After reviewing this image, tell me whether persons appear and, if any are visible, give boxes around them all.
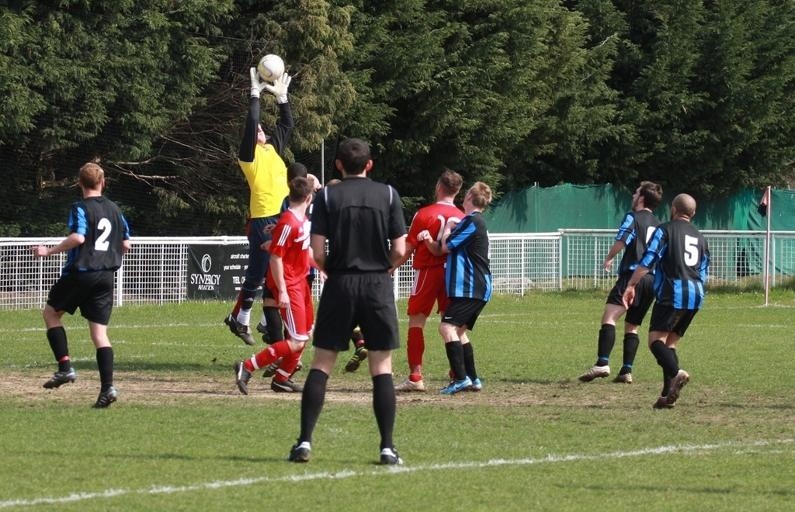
[263,162,317,379]
[32,164,131,410]
[232,178,320,396]
[223,66,295,348]
[622,193,710,409]
[390,170,467,392]
[324,170,368,375]
[578,180,663,384]
[288,136,406,466]
[416,181,492,394]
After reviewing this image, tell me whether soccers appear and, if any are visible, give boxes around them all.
[257,54,285,81]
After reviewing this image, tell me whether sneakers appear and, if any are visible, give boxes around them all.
[271,376,303,393]
[440,376,473,394]
[659,368,690,404]
[379,442,399,465]
[652,396,674,410]
[288,438,313,463]
[468,373,484,391]
[576,365,610,382]
[43,366,77,390]
[234,321,257,347]
[224,314,237,334]
[232,360,252,396]
[613,368,633,385]
[91,384,119,409]
[262,365,277,378]
[344,344,369,372]
[261,333,282,344]
[393,376,427,393]
[255,323,268,333]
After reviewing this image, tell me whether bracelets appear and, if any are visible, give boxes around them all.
[249,86,262,92]
